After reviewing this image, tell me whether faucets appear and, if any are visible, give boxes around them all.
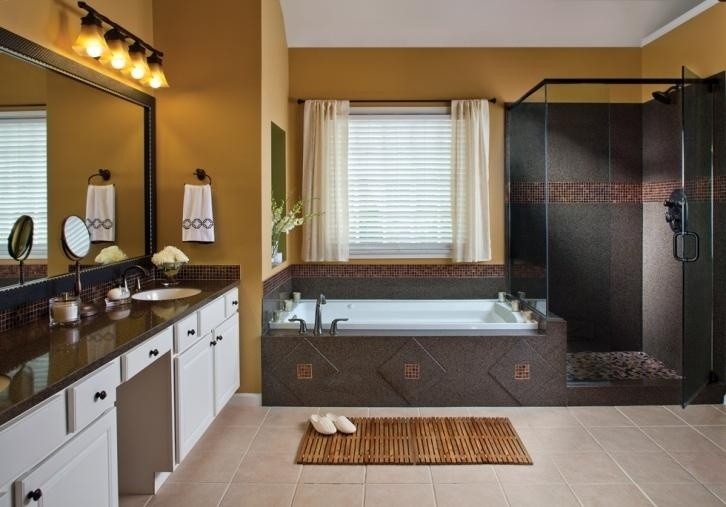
[313,294,326,335]
[122,264,149,294]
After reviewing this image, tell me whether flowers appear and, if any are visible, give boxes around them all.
[272,190,325,255]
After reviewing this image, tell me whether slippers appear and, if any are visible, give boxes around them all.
[326,412,357,433]
[310,414,337,434]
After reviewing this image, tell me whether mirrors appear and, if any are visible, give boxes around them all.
[7,215,33,285]
[61,215,98,317]
[0,26,156,311]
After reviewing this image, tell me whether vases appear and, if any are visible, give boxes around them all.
[271,241,278,267]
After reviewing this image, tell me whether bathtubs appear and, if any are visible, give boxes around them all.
[269,299,539,330]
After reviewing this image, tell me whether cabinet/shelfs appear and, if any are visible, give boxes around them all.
[0,280,240,507]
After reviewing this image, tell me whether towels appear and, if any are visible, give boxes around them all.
[182,183,215,242]
[86,183,116,241]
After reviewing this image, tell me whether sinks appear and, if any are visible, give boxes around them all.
[131,288,202,300]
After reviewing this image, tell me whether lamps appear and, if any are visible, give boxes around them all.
[71,13,113,58]
[98,27,135,75]
[138,52,169,88]
[120,41,153,85]
